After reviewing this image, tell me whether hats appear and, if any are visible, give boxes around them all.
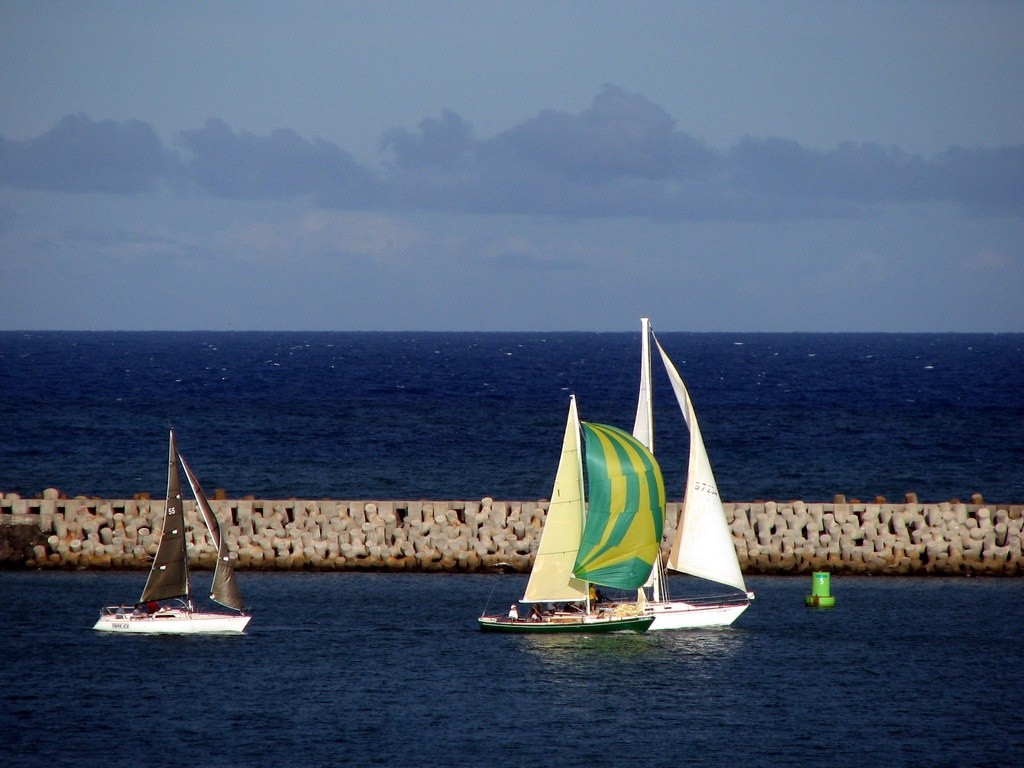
[511,605,516,609]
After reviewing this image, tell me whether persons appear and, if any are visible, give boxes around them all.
[133,604,141,615]
[508,583,612,620]
[117,604,125,614]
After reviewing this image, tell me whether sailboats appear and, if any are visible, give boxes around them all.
[94,427,251,636]
[478,318,756,634]
